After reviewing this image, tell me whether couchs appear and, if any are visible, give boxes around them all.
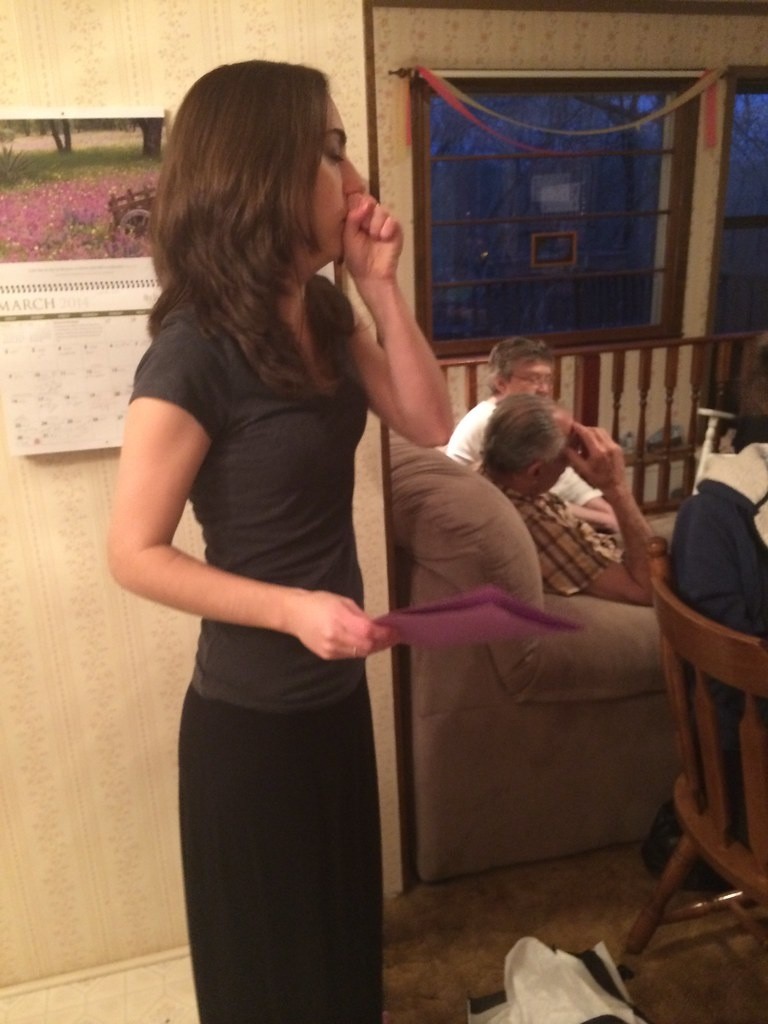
[386,431,687,882]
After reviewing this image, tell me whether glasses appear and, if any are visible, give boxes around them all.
[510,374,557,386]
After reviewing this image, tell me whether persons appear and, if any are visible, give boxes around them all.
[446,335,622,535]
[106,62,455,1023]
[470,392,675,608]
[663,329,768,878]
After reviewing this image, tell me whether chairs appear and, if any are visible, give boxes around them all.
[626,538,767,957]
